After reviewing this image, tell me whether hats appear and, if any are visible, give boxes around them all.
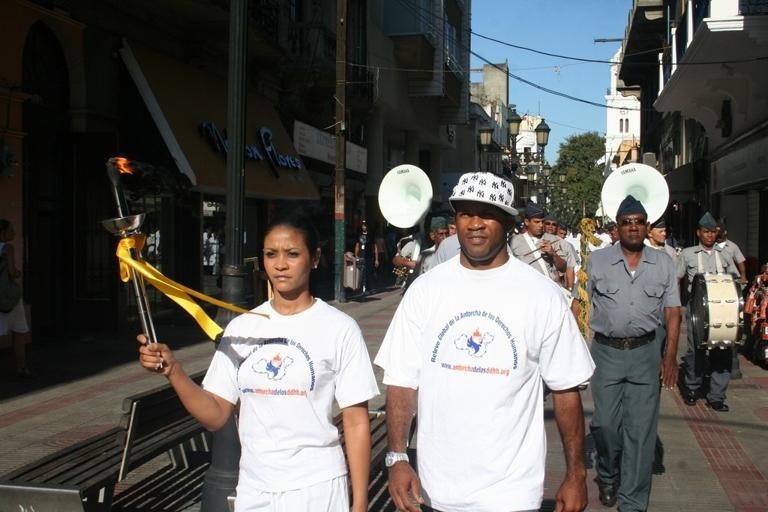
[651,217,666,228]
[617,195,647,217]
[697,211,718,231]
[448,172,520,216]
[525,202,567,230]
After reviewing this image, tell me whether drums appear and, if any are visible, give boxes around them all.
[690,272,748,351]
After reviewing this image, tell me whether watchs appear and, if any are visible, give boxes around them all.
[383,448,411,466]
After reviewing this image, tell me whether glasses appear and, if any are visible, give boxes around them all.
[621,218,644,224]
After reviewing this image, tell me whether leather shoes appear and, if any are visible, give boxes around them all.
[686,391,696,405]
[712,403,728,410]
[601,492,617,506]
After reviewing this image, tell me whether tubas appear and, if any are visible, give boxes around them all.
[601,163,670,226]
[377,163,433,228]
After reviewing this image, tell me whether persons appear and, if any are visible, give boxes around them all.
[136,213,382,511]
[353,218,378,295]
[388,205,749,413]
[371,170,599,511]
[585,195,683,511]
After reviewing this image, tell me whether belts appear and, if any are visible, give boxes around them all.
[593,332,656,350]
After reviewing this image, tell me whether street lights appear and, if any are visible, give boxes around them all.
[475,106,551,181]
[533,161,576,219]
